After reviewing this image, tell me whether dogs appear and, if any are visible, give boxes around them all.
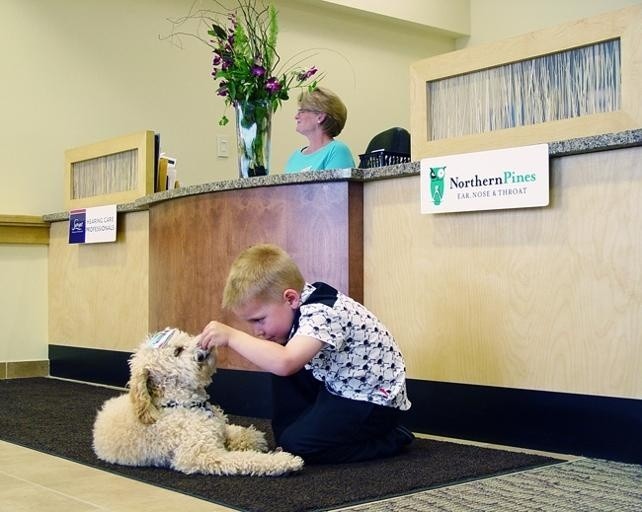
[90,325,305,479]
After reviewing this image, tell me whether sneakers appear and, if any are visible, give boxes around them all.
[378,425,415,455]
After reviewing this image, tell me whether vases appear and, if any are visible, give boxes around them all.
[234,99,270,178]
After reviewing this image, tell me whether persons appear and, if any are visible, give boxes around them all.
[199,245,415,462]
[284,90,355,173]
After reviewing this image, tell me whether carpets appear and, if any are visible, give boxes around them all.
[320,429,642,512]
[0,372,586,512]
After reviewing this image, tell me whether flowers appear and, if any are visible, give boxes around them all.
[157,0,324,175]
